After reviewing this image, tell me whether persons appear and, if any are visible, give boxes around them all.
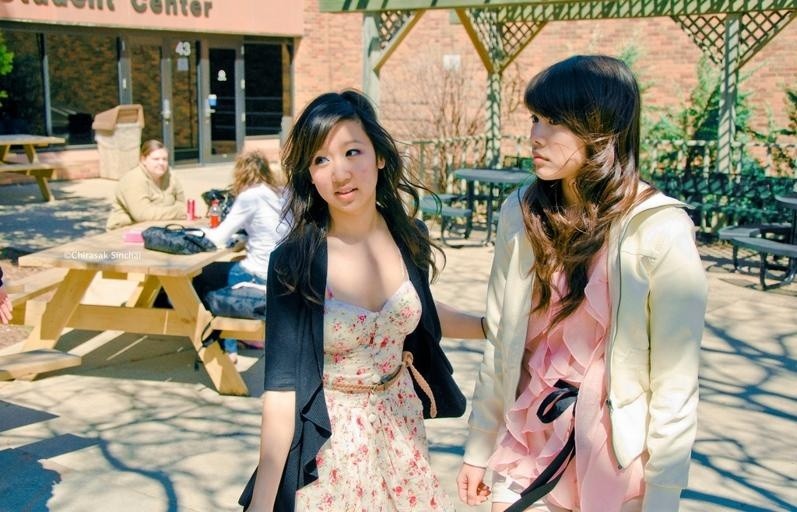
[101,134,190,309]
[183,145,295,369]
[0,266,13,325]
[453,51,711,512]
[236,87,467,511]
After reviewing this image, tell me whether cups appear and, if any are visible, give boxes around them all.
[186,199,194,220]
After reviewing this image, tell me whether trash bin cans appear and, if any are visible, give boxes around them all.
[92,104,145,180]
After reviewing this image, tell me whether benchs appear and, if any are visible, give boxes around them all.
[4,267,72,324]
[719,218,796,289]
[412,192,473,250]
[210,313,265,341]
[1,163,56,177]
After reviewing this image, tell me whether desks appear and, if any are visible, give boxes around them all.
[18,214,252,398]
[452,166,533,249]
[0,134,66,202]
[771,193,796,285]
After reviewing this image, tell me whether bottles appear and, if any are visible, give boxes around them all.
[207,200,220,228]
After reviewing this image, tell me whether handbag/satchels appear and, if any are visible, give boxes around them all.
[141,223,216,255]
[203,281,268,319]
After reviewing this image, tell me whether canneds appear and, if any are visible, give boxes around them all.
[187,199,195,221]
